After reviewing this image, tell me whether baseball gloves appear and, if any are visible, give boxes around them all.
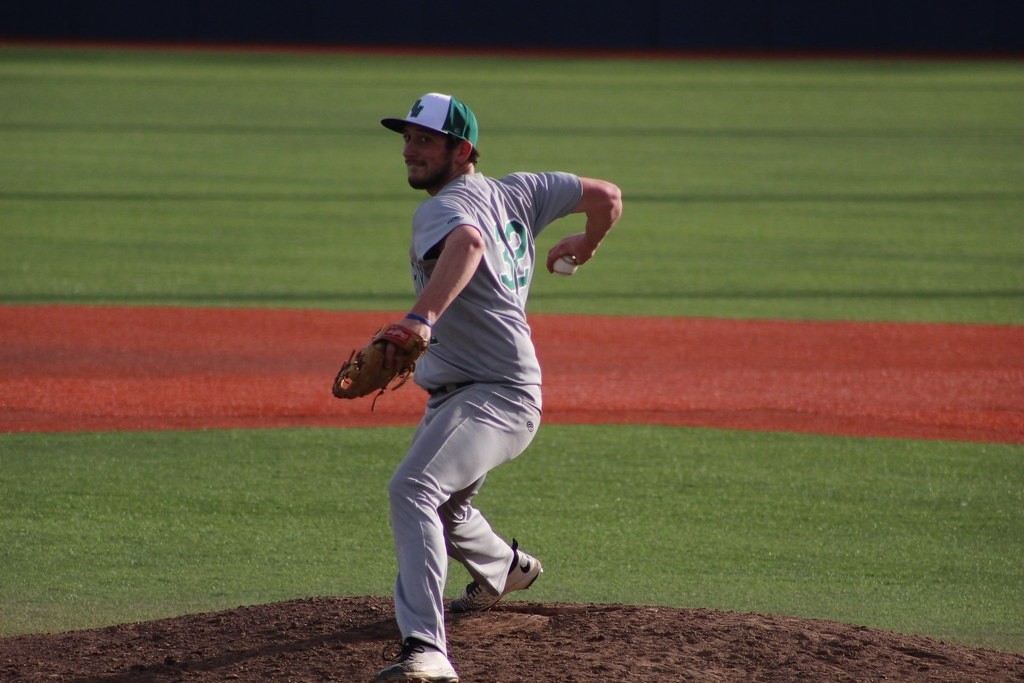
[330,322,429,411]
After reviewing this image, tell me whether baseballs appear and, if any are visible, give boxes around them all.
[553,254,580,278]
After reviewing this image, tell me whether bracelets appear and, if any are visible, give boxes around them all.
[405,314,435,328]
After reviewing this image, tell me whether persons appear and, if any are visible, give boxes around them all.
[331,90,623,683]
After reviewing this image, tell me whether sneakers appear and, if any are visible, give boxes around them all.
[447,539,543,614]
[377,642,459,682]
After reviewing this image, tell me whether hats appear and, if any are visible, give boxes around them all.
[381,91,479,149]
